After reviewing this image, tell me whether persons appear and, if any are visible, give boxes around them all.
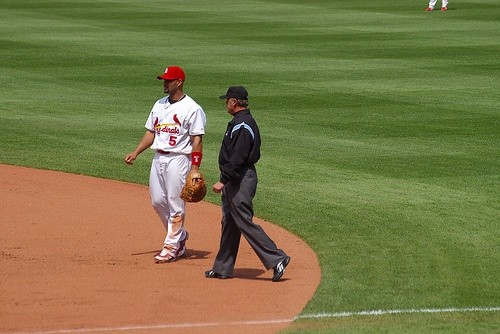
[204,86,291,283]
[425,0,448,11]
[123,65,206,265]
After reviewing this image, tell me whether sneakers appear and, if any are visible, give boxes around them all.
[154,246,182,263]
[272,256,290,281]
[176,231,189,257]
[205,270,227,279]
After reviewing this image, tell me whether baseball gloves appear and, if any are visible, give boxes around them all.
[179,172,207,203]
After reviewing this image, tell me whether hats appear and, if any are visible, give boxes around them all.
[220,86,249,100]
[157,66,185,81]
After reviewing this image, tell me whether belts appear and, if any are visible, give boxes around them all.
[157,150,170,154]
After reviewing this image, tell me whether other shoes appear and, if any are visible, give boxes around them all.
[426,7,435,11]
[441,7,447,11]
